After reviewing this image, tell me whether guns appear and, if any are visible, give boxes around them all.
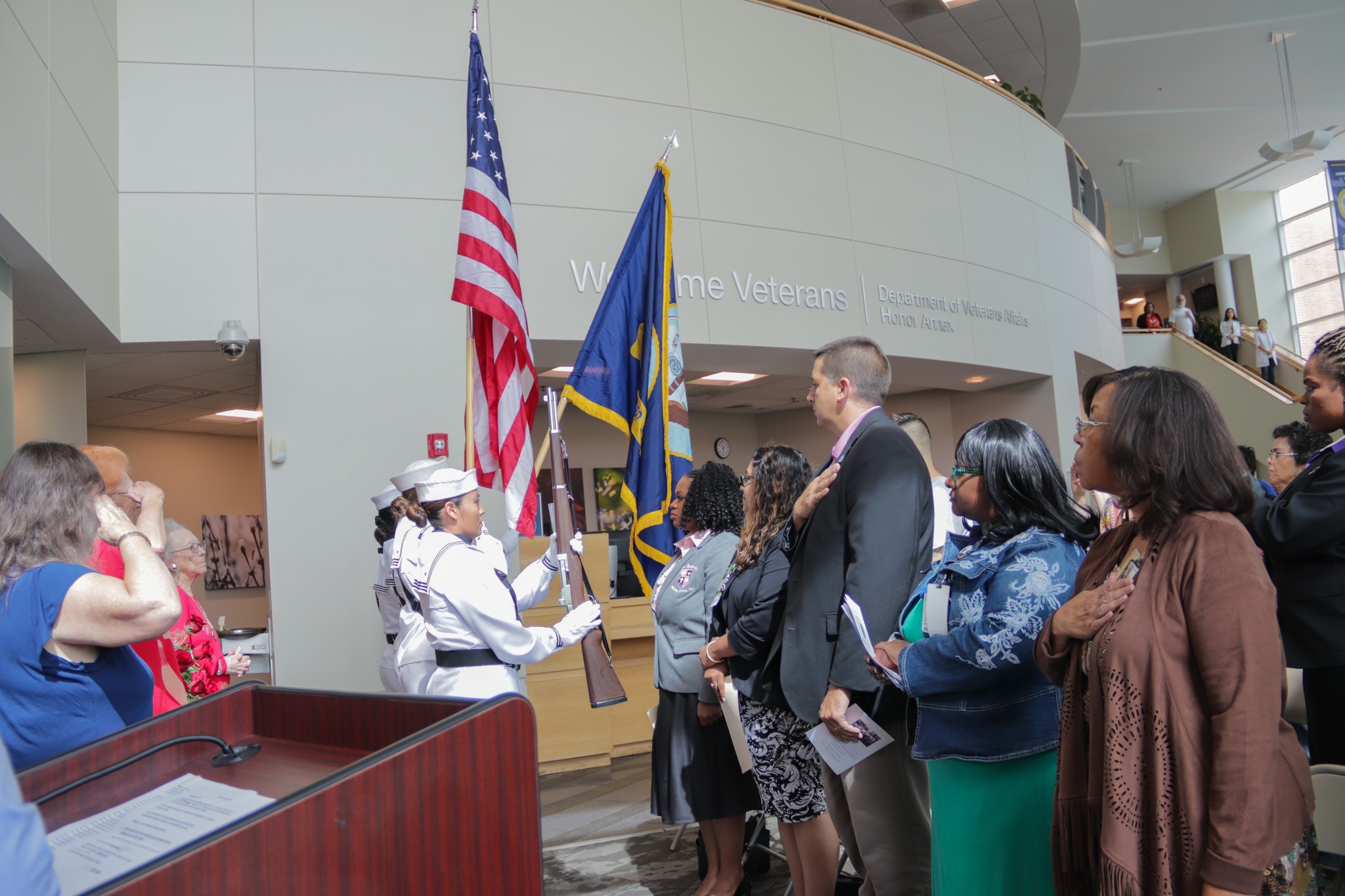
[542,385,630,710]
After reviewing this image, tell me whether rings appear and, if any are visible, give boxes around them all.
[711,683,718,688]
[834,726,840,731]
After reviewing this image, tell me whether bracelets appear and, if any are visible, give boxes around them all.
[117,530,150,548]
[705,639,724,663]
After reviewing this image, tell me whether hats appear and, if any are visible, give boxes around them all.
[390,455,447,492]
[370,484,402,510]
[414,468,479,503]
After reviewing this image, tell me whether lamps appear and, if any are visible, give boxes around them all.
[1257,33,1335,163]
[1113,158,1162,259]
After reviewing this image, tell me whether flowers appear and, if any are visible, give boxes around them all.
[171,614,230,704]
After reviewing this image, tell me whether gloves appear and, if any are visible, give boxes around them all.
[547,532,583,567]
[552,601,602,645]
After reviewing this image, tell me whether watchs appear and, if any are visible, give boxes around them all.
[216,658,223,675]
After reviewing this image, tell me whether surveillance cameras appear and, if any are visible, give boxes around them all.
[215,320,250,361]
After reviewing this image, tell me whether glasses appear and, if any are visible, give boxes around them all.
[952,464,984,483]
[170,540,207,553]
[1076,416,1116,437]
[1266,452,1297,459]
[740,475,757,487]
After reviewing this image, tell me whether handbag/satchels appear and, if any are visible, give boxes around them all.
[695,811,771,882]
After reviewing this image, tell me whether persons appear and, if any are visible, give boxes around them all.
[0,442,182,773]
[1220,307,1243,365]
[1254,319,1280,387]
[73,445,188,718]
[152,515,252,700]
[1136,304,1162,334]
[1168,293,1198,341]
[646,326,1345,896]
[371,457,602,697]
[0,736,60,896]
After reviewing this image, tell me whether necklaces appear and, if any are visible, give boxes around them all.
[175,583,217,639]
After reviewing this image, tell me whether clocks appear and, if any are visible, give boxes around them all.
[714,437,730,459]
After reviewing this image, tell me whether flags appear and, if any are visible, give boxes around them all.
[448,30,541,541]
[564,161,692,608]
